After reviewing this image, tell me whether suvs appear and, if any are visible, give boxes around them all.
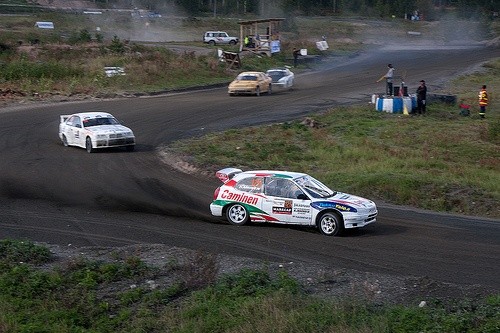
[202,30,239,47]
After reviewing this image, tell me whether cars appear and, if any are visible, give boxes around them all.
[266,67,295,89]
[209,167,379,237]
[227,71,273,97]
[58,111,136,154]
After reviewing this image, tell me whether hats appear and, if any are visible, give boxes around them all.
[419,80,425,82]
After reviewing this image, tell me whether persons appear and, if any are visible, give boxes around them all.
[385,63,395,97]
[293,48,302,67]
[416,80,428,115]
[410,9,419,21]
[267,27,272,39]
[245,36,249,47]
[399,82,409,96]
[479,85,487,118]
